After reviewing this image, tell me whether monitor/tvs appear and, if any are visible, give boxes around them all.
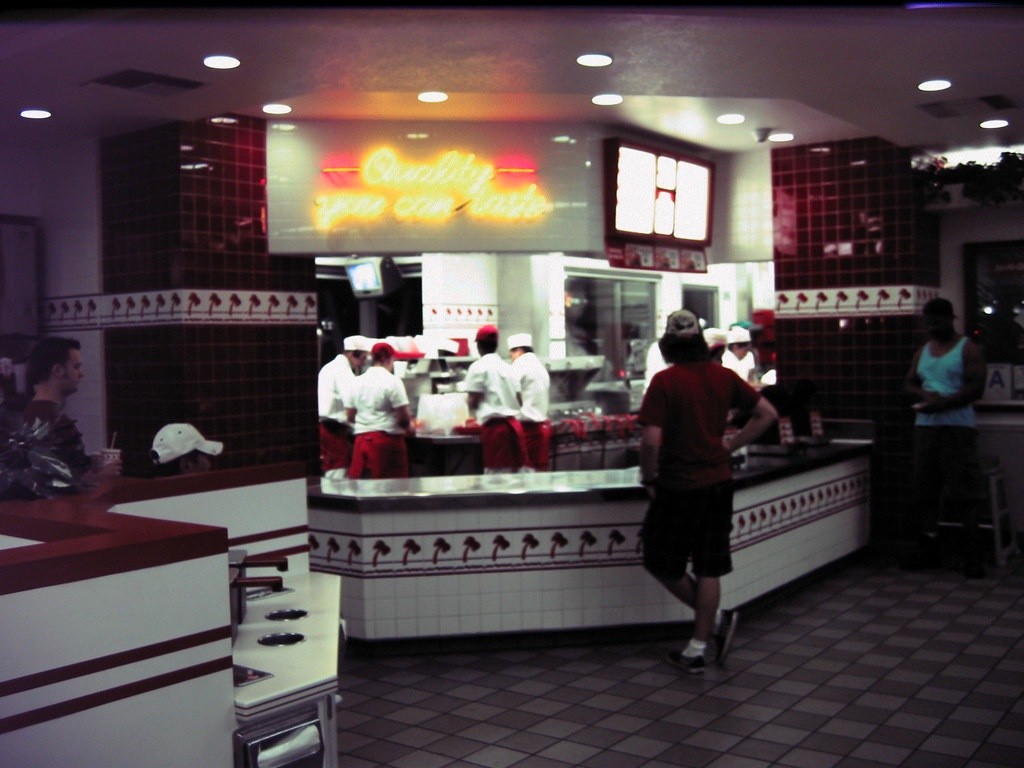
[344,255,401,299]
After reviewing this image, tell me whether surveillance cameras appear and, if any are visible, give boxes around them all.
[752,128,772,143]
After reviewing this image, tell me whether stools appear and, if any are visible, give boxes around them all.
[934,454,1010,563]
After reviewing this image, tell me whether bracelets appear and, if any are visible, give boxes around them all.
[640,471,658,486]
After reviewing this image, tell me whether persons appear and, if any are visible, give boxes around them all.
[463,325,530,474]
[637,319,778,674]
[898,298,987,578]
[319,335,373,470]
[642,320,777,421]
[149,422,223,477]
[343,343,416,479]
[506,334,550,472]
[21,337,122,479]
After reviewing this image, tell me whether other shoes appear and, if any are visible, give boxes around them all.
[713,610,739,664]
[665,645,707,674]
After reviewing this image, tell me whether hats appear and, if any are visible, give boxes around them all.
[149,423,225,464]
[475,325,499,340]
[664,309,700,337]
[702,327,726,350]
[726,325,752,344]
[370,343,394,357]
[922,298,960,320]
[343,334,371,352]
[506,328,534,350]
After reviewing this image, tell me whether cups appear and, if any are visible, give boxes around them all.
[90,448,121,478]
